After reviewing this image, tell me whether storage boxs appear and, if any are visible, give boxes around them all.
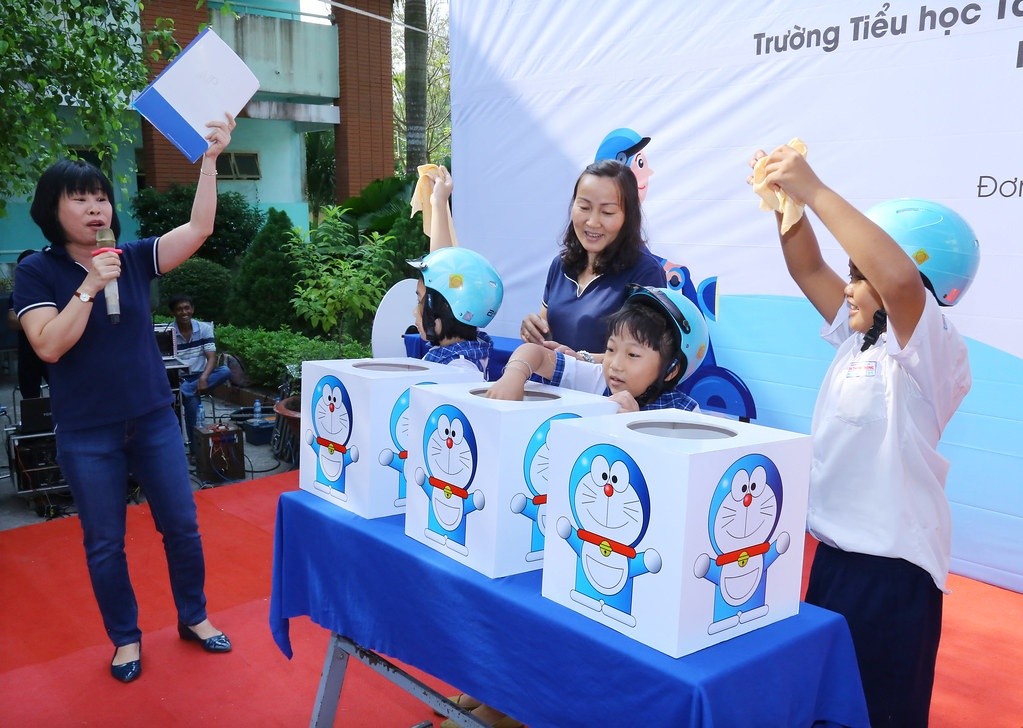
[541,407,815,657]
[403,379,621,580]
[300,356,484,519]
[245,417,276,446]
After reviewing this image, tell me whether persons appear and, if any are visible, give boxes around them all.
[8,250,49,398]
[406,165,524,728]
[483,284,709,413]
[167,295,232,463]
[520,160,665,363]
[13,112,236,683]
[747,145,981,728]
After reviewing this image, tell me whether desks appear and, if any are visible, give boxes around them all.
[271,489,872,728]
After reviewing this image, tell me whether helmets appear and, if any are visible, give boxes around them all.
[624,283,710,390]
[862,198,981,308]
[405,246,504,328]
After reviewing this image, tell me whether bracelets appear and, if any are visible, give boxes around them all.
[502,366,527,381]
[505,359,532,380]
[579,351,594,363]
[201,169,218,176]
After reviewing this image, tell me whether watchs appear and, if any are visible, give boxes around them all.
[74,292,94,303]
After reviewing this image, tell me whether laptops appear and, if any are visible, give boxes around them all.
[15,398,53,434]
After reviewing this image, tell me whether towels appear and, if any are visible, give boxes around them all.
[409,163,460,247]
[753,136,808,236]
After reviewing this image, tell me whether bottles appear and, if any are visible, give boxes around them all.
[253,399,261,421]
[196,405,205,429]
[275,397,281,404]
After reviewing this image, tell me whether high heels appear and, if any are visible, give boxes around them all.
[177,619,232,652]
[111,639,140,683]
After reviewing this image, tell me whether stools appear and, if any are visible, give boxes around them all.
[0,405,13,480]
[201,393,216,424]
[12,384,49,425]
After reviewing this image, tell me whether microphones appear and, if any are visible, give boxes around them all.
[95,228,121,326]
